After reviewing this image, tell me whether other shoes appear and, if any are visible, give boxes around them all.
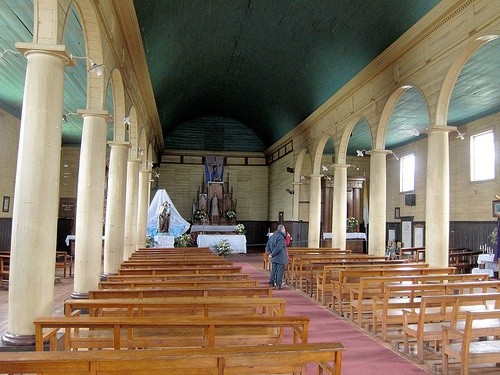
[269,287,283,290]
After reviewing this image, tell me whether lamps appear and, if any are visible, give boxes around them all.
[322,164,331,171]
[301,175,306,180]
[287,167,295,173]
[457,129,466,140]
[146,159,160,178]
[70,54,106,77]
[138,147,144,156]
[123,115,131,126]
[63,113,79,123]
[356,149,366,156]
[352,165,359,172]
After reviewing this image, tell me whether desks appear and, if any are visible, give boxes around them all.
[323,233,366,253]
[196,234,247,254]
[191,225,237,241]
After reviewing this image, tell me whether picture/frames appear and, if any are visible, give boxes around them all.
[395,207,401,219]
[3,196,10,212]
[492,200,500,217]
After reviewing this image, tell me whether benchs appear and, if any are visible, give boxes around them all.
[65,235,105,260]
[0,247,500,375]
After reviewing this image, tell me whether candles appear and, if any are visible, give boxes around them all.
[480,244,493,254]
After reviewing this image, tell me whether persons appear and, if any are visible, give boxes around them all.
[272,223,290,247]
[266,224,288,289]
[212,192,219,215]
[157,201,174,234]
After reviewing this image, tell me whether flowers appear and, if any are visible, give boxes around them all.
[347,217,358,229]
[235,224,245,234]
[175,234,194,247]
[224,210,236,220]
[195,209,207,221]
[211,239,233,256]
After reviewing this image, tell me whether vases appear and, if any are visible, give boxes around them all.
[349,227,353,233]
